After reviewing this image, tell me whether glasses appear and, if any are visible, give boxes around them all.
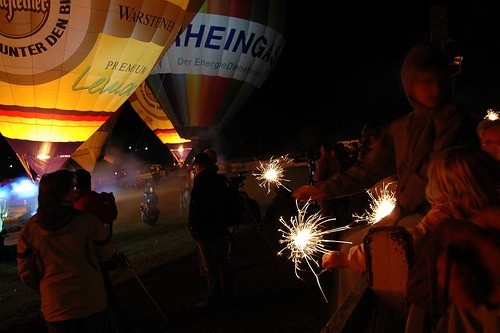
[64,186,77,193]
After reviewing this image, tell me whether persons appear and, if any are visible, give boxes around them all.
[17,169,116,333]
[291,36,500,333]
[68,168,118,224]
[187,148,233,308]
[263,185,295,222]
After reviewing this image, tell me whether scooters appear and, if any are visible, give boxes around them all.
[140,196,159,226]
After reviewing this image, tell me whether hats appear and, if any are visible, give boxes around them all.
[190,152,212,166]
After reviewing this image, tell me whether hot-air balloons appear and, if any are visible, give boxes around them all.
[143,0,286,181]
[126,80,197,174]
[0,0,206,188]
